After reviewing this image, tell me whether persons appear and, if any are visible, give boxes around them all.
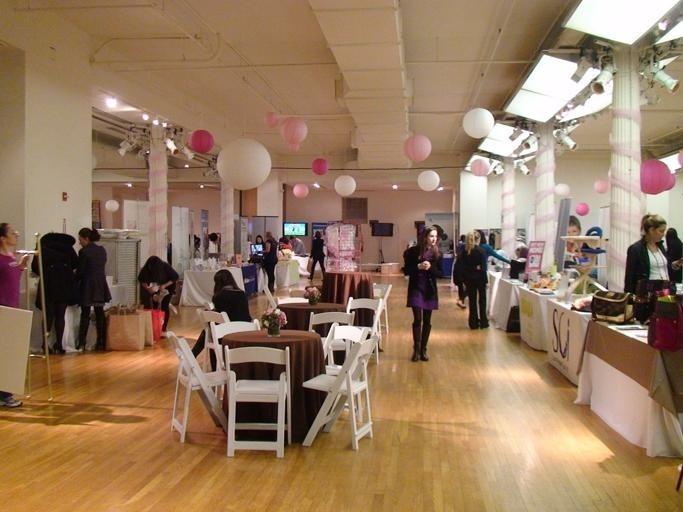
[138,256,180,332]
[255,232,278,292]
[476,229,511,272]
[665,227,683,282]
[453,234,468,308]
[255,234,265,248]
[0,222,30,408]
[567,215,581,236]
[75,227,112,351]
[460,230,490,329]
[403,226,444,362]
[191,269,252,372]
[31,232,78,354]
[307,231,326,281]
[624,213,683,324]
[290,235,306,255]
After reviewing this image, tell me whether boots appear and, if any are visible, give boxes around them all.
[76,326,88,350]
[95,328,103,350]
[412,324,420,361]
[421,325,431,360]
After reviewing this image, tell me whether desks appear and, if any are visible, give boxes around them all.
[278,302,349,365]
[494,277,526,332]
[323,271,374,327]
[586,319,679,429]
[223,331,330,443]
[547,298,590,387]
[517,284,559,351]
[183,263,266,307]
[275,259,299,288]
[488,269,502,319]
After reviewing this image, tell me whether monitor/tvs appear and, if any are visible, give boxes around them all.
[283,222,308,236]
[249,243,264,257]
[371,222,394,236]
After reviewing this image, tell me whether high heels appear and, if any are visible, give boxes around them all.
[53,343,65,354]
[308,277,313,281]
[42,344,51,354]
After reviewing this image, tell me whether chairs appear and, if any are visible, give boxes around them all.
[209,319,261,408]
[195,308,231,401]
[325,322,369,433]
[223,345,293,458]
[302,334,381,451]
[373,281,392,351]
[307,311,356,352]
[165,331,237,444]
[345,297,383,365]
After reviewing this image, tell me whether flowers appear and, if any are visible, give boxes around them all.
[303,287,323,306]
[261,309,288,336]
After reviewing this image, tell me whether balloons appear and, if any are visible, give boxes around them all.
[555,183,570,197]
[594,180,608,193]
[641,158,675,194]
[462,108,494,139]
[470,158,490,177]
[311,158,328,175]
[217,136,272,191]
[404,134,432,162]
[417,170,440,191]
[280,118,308,152]
[334,175,356,197]
[576,202,589,216]
[264,111,279,128]
[189,129,214,153]
[293,183,309,199]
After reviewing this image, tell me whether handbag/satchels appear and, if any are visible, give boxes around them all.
[648,290,683,351]
[589,292,636,326]
[106,303,144,351]
[134,311,155,347]
[143,297,165,343]
[248,252,264,264]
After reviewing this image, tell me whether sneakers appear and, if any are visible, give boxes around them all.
[0,396,22,407]
[456,301,466,309]
[480,324,489,329]
